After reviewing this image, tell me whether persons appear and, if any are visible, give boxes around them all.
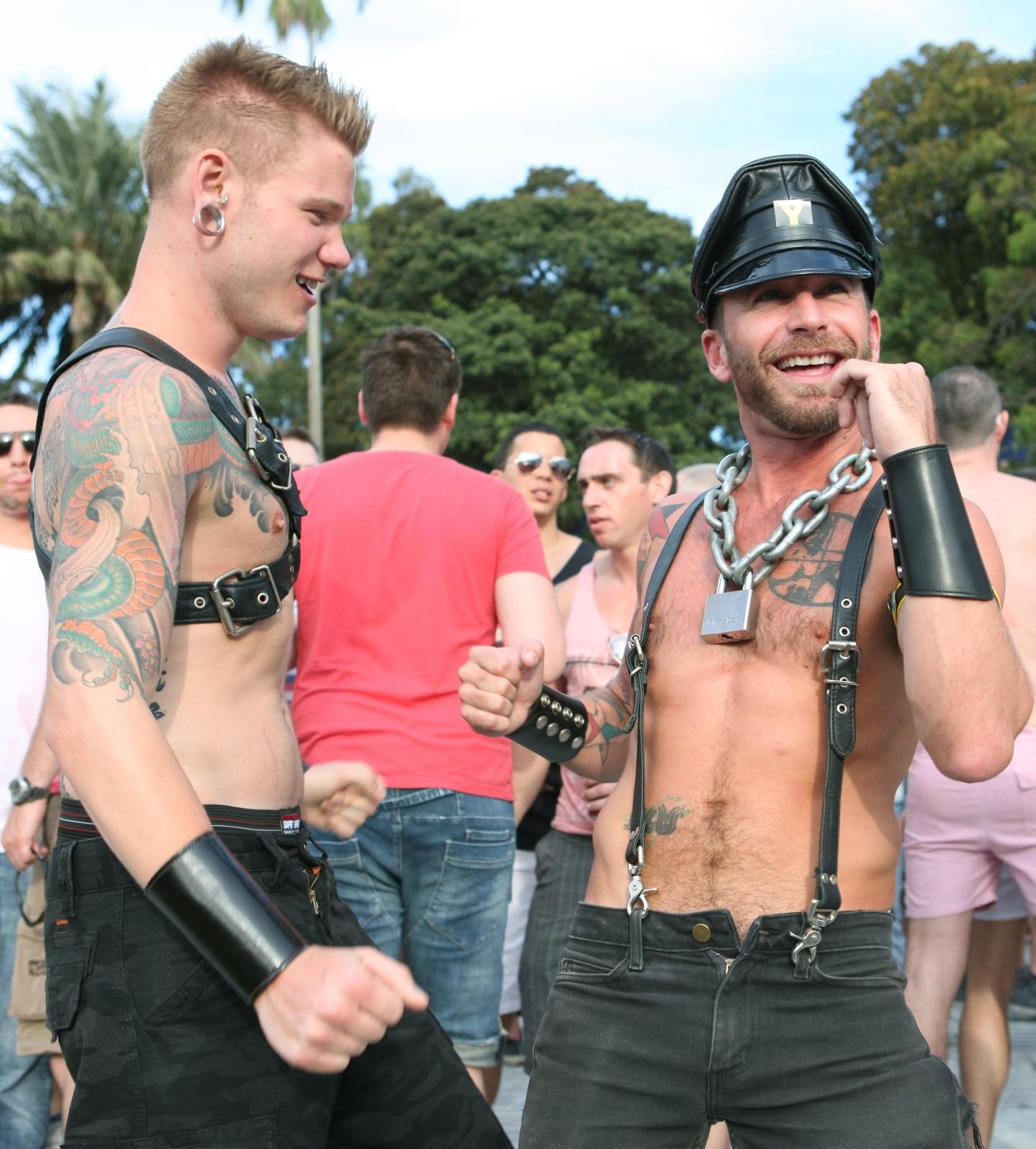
[459,156,1035,1149]
[0,326,1036,1149]
[30,41,516,1149]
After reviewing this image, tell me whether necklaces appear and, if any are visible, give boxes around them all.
[698,443,879,647]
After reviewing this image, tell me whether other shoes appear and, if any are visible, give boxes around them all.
[45,1114,63,1146]
[951,959,1036,1021]
[501,1028,525,1067]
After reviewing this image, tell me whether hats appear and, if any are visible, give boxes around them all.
[691,154,885,328]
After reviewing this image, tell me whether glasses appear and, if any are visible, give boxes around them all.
[0,429,33,454]
[507,452,573,482]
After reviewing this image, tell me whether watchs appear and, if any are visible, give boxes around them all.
[8,774,49,806]
